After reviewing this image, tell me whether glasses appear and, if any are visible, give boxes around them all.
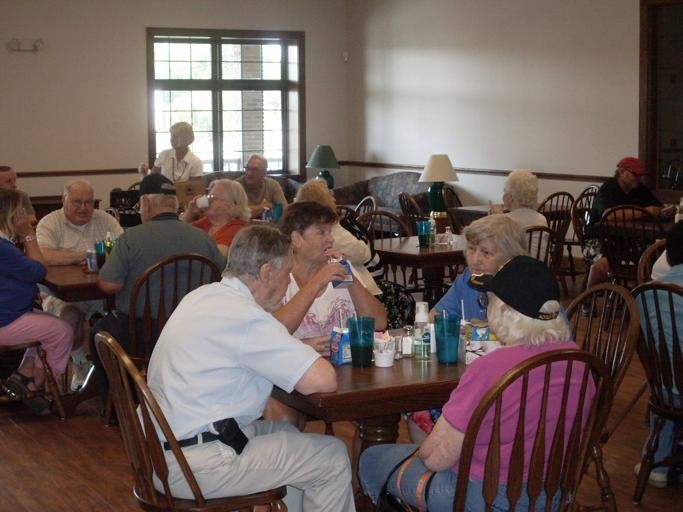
[626,169,640,177]
[243,167,266,174]
[207,195,235,204]
[477,293,489,309]
[72,199,93,208]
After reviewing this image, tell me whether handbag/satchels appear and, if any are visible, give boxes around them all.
[376,448,436,512]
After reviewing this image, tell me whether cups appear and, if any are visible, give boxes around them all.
[373,348,395,367]
[95,241,106,270]
[196,195,209,208]
[347,316,375,369]
[416,221,432,248]
[437,233,450,245]
[433,314,461,363]
[86,250,97,271]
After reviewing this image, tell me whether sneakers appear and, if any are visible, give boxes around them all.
[634,463,668,488]
[71,361,90,391]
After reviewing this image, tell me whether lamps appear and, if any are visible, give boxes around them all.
[305,144,341,190]
[417,155,459,218]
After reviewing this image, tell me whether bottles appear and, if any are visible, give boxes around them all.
[444,226,452,245]
[401,326,415,358]
[414,302,431,362]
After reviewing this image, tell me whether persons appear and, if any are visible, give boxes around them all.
[232,156,287,218]
[136,224,356,508]
[404,214,575,445]
[581,158,671,307]
[488,170,548,261]
[0,165,37,250]
[179,178,249,259]
[264,203,388,444]
[359,257,595,507]
[2,190,72,417]
[95,172,224,424]
[634,224,683,488]
[140,120,201,198]
[37,183,125,391]
[295,177,413,330]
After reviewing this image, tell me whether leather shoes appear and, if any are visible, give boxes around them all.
[582,303,597,317]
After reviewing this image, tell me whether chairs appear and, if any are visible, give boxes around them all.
[102,253,221,426]
[523,226,563,273]
[335,206,355,225]
[625,283,683,504]
[355,196,377,232]
[552,184,599,283]
[95,330,292,512]
[454,348,615,512]
[442,184,463,236]
[398,190,425,234]
[600,204,666,332]
[0,340,68,421]
[566,283,640,512]
[572,192,603,299]
[356,210,419,304]
[103,207,119,225]
[537,192,575,295]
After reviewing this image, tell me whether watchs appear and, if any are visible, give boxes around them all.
[18,235,36,243]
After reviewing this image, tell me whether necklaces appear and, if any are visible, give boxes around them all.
[171,155,187,184]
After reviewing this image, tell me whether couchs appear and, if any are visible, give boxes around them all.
[200,171,304,206]
[335,172,463,239]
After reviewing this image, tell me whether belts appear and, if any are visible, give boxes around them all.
[163,432,221,451]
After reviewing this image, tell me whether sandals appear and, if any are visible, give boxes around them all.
[6,370,50,413]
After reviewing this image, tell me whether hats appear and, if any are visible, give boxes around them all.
[617,158,645,174]
[468,256,561,320]
[130,173,177,205]
